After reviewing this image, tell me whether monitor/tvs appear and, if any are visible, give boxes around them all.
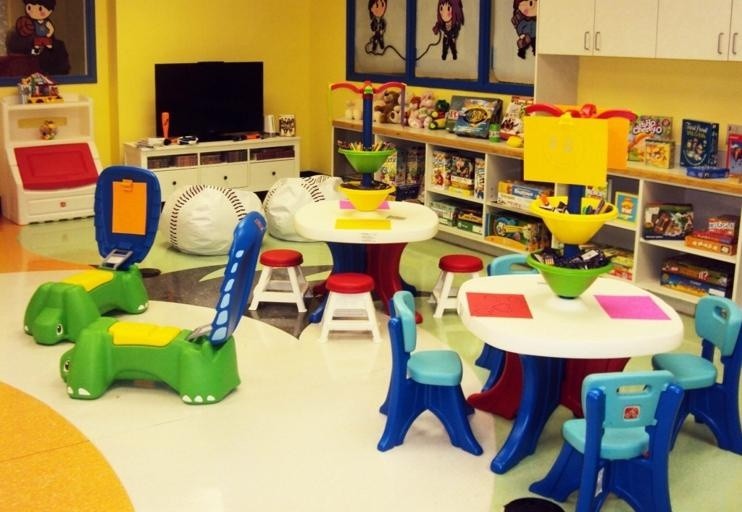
[155,61,264,142]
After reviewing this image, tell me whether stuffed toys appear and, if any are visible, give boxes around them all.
[345,91,450,130]
